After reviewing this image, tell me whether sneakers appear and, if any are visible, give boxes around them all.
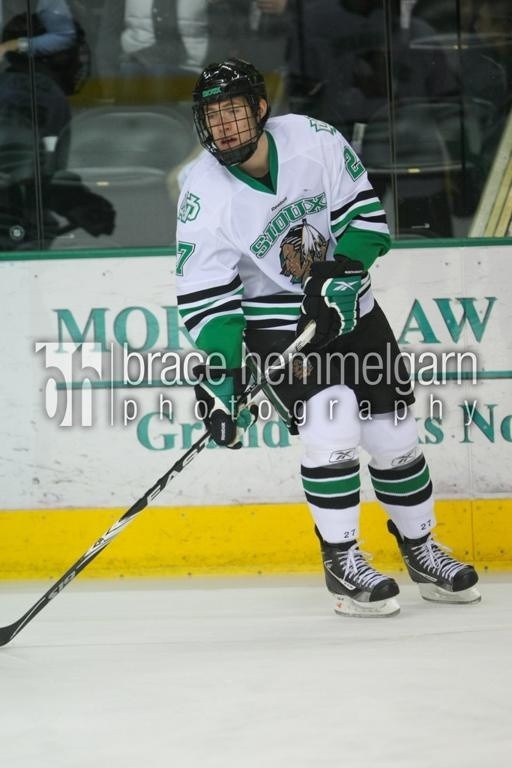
[323,540,399,603]
[404,533,478,591]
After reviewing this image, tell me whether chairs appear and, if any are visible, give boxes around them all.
[0,2,512,254]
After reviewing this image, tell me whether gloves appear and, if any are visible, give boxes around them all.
[297,254,371,349]
[193,365,258,449]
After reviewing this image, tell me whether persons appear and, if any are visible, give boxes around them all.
[1,1,512,252]
[172,59,481,602]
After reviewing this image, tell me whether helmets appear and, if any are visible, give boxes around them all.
[192,57,271,167]
